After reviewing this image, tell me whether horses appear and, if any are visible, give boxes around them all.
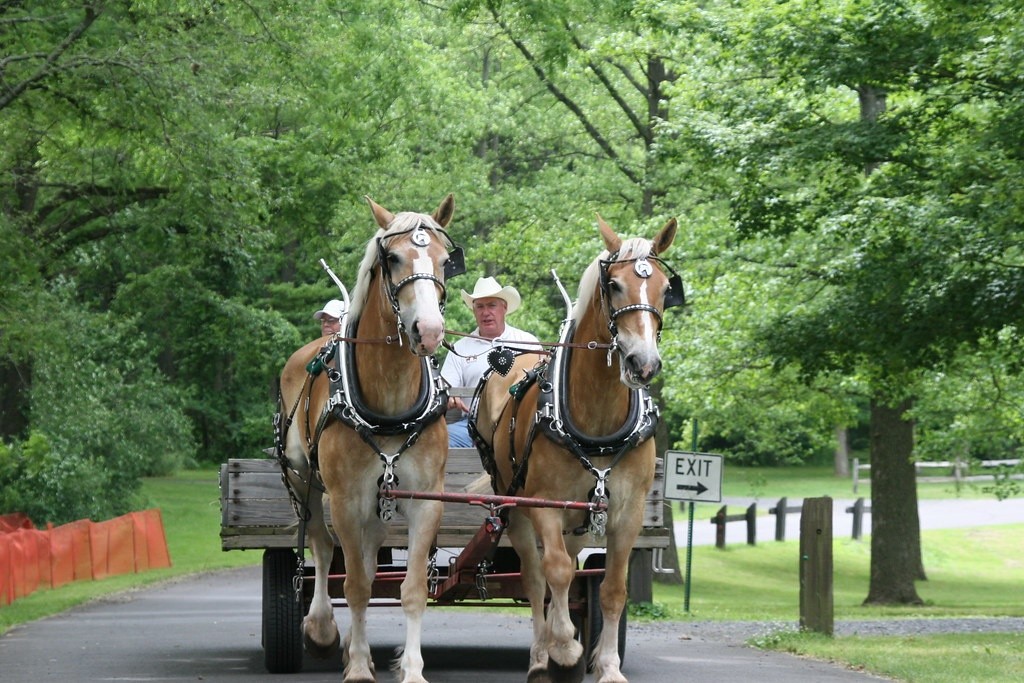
[278,195,455,683]
[469,211,678,683]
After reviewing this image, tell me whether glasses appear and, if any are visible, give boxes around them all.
[320,317,337,325]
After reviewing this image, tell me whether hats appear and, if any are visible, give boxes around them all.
[460,276,521,316]
[313,300,344,319]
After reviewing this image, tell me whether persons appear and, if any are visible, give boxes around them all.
[435,276,541,446]
[313,299,346,337]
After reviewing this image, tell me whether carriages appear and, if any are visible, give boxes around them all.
[217,194,682,664]
